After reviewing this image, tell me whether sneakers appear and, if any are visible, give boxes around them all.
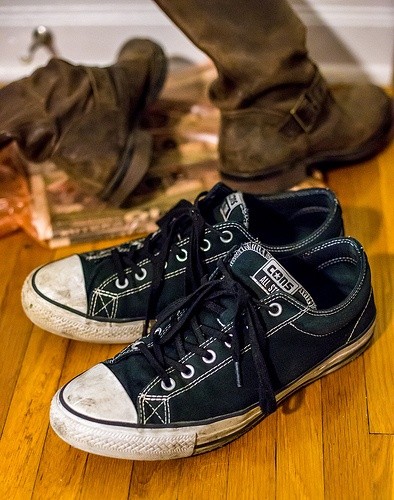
[21,181,345,344]
[49,237,377,461]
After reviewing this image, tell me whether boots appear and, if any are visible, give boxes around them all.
[0,39,166,210]
[156,0,393,194]
